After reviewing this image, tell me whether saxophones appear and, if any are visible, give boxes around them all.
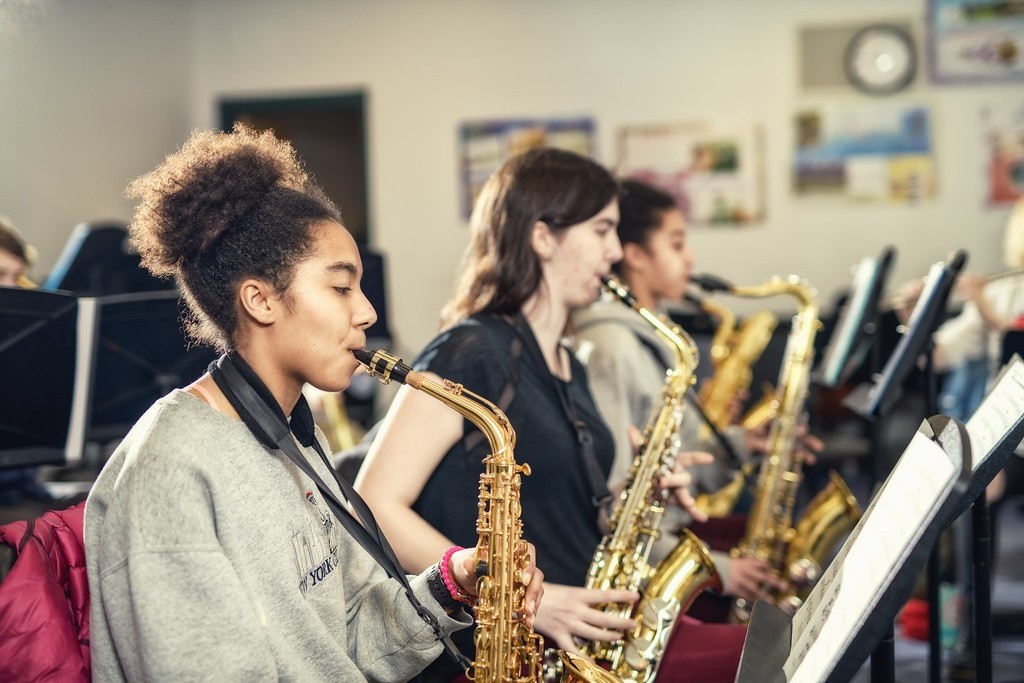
[681,290,780,518]
[687,271,865,627]
[570,273,727,683]
[351,346,621,683]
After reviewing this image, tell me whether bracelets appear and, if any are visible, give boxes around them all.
[440,547,478,607]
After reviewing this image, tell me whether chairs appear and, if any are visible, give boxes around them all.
[0,501,93,683]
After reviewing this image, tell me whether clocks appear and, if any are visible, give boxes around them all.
[842,24,916,97]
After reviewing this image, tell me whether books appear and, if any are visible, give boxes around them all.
[782,361,1024,683]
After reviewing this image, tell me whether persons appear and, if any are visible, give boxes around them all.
[558,178,826,607]
[0,218,36,289]
[345,146,713,665]
[83,123,543,683]
[826,202,1024,644]
